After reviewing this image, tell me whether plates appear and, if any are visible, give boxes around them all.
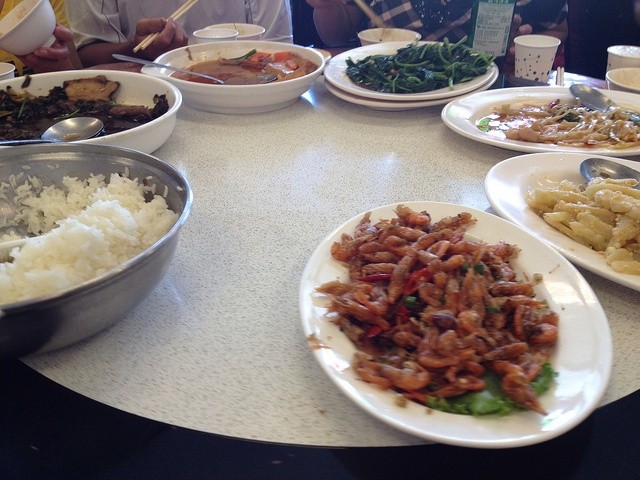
[326,41,496,100]
[327,56,501,112]
[300,201,612,446]
[484,151,640,290]
[439,85,638,155]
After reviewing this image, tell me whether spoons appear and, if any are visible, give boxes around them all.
[0,117,103,147]
[579,158,639,195]
[570,84,640,123]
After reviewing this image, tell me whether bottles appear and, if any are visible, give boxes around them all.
[466,0,516,71]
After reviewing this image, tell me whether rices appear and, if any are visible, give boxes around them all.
[0,160,183,304]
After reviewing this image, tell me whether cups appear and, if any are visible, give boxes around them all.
[193,29,238,40]
[513,35,560,83]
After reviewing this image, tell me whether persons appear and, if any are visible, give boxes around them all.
[1,0,85,73]
[64,0,294,68]
[305,0,570,66]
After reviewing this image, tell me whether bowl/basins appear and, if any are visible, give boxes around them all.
[152,39,324,115]
[358,27,420,41]
[0,69,182,155]
[603,67,638,93]
[0,143,193,360]
[210,22,266,40]
[1,1,58,55]
[0,62,16,80]
[609,44,640,67]
